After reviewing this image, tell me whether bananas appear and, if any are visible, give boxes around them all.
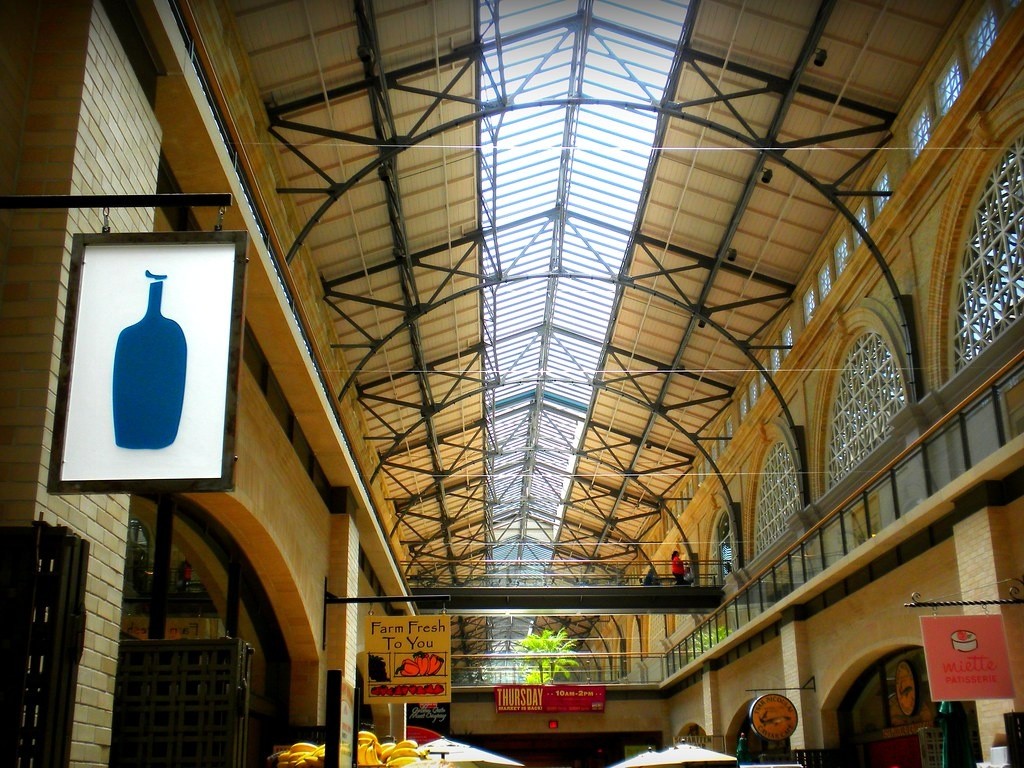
[357,730,431,768]
[276,742,326,768]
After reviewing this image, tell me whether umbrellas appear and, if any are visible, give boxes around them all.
[736,732,751,762]
[418,738,524,766]
[938,701,975,768]
[609,738,737,768]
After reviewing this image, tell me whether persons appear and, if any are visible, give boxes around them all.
[643,569,659,586]
[671,550,683,583]
[675,567,693,586]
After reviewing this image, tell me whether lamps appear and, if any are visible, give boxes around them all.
[762,167,773,183]
[393,247,405,262]
[356,44,373,61]
[587,516,617,573]
[646,442,652,449]
[813,47,828,66]
[622,493,629,501]
[698,320,706,327]
[727,247,737,261]
[378,164,392,182]
